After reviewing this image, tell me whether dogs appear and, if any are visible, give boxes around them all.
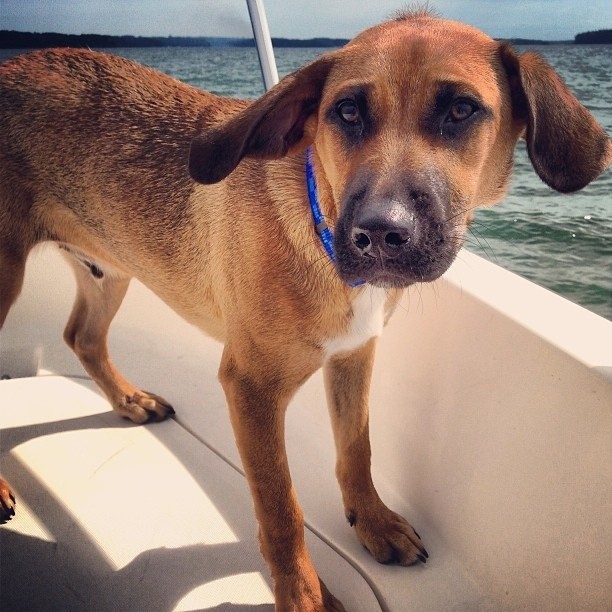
[0,1,611,612]
